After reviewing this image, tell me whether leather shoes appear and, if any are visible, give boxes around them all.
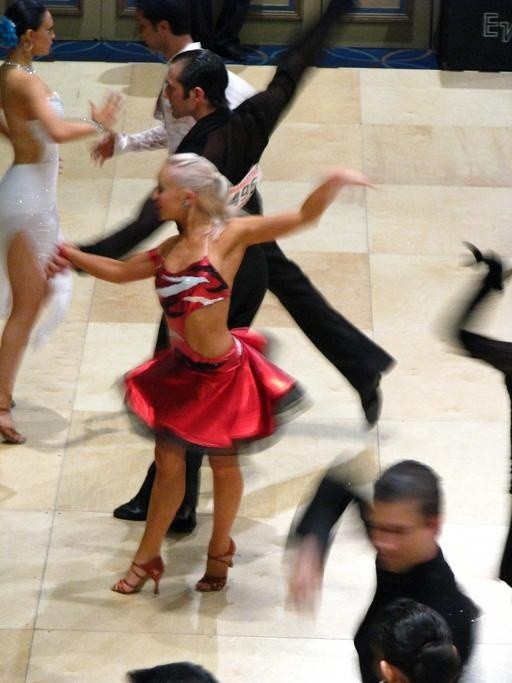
[170,471,198,532]
[113,461,157,521]
[357,357,393,423]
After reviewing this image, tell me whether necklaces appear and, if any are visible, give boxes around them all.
[4,57,37,79]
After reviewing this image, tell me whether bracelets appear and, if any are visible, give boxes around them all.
[90,120,104,129]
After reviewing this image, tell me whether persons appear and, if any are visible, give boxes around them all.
[46,0,365,537]
[452,240,512,588]
[86,0,397,427]
[55,150,380,598]
[1,0,133,451]
[280,445,481,682]
[368,594,462,681]
[170,0,257,64]
[127,660,219,683]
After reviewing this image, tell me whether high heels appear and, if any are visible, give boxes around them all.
[195,536,236,591]
[111,556,164,594]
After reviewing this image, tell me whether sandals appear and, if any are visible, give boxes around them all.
[0,407,27,444]
